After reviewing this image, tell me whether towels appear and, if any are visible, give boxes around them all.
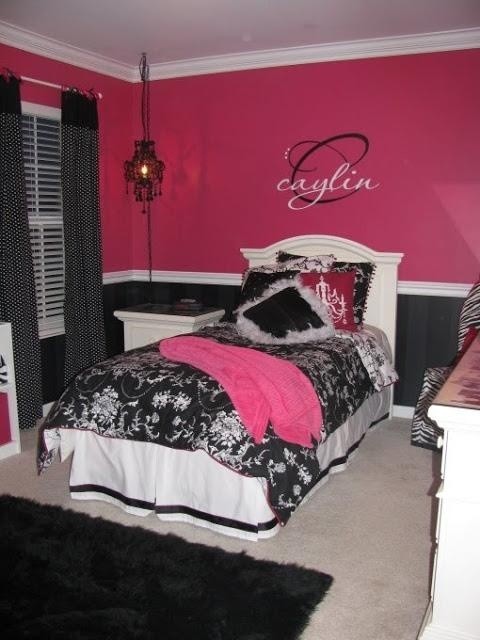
[159,336,321,448]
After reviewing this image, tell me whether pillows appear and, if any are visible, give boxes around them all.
[232,250,376,346]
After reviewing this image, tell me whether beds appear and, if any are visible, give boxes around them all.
[36,234,405,543]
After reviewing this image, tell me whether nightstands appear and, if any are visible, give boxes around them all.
[114,302,226,352]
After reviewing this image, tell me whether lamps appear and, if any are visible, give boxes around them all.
[123,54,165,214]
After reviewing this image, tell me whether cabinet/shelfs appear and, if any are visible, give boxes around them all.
[415,331,480,639]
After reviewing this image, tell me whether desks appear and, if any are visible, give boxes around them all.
[0,322,22,461]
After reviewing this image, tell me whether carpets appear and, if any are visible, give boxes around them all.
[0,493,333,639]
